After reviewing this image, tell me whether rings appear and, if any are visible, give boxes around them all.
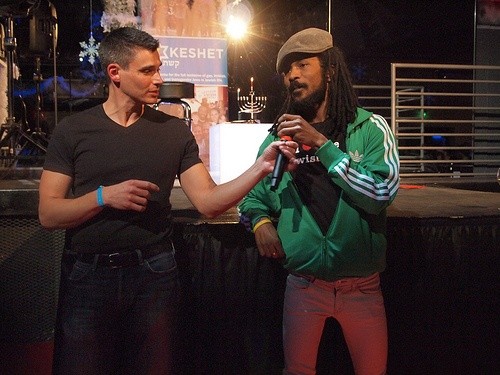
[272,251,278,257]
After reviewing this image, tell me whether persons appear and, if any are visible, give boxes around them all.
[238,28,399,375]
[39,27,301,375]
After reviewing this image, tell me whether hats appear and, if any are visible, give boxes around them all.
[276,28,332,75]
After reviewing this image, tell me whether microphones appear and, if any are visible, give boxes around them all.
[270,133,293,190]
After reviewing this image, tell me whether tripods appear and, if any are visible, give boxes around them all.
[0,16,50,168]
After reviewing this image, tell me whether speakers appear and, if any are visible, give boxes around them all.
[21,0,58,58]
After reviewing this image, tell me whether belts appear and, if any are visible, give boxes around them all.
[63,240,172,266]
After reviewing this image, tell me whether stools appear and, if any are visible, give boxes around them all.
[152,80,197,132]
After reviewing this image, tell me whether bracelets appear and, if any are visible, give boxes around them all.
[97,185,108,209]
[253,219,272,233]
